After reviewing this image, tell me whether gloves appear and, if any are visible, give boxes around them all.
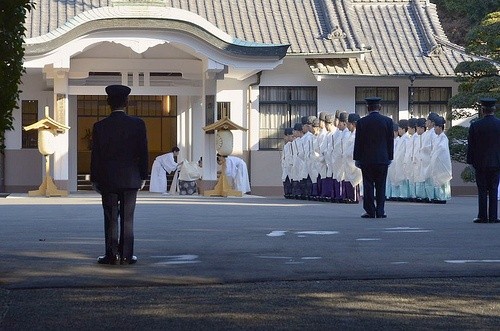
[468,164,475,172]
[387,160,391,167]
[355,160,360,168]
[138,180,146,191]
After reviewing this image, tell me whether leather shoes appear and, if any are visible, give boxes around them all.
[97,254,118,265]
[120,255,137,265]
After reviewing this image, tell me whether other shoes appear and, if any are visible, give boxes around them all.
[489,217,500,223]
[473,217,488,223]
[376,214,387,218]
[361,213,375,218]
[283,192,447,205]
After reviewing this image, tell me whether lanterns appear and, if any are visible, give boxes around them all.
[217,130,233,158]
[38,128,56,157]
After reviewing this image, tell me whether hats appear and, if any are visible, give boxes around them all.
[398,120,408,128]
[415,118,426,127]
[434,116,444,125]
[428,112,439,122]
[364,97,382,105]
[406,118,417,127]
[480,98,497,107]
[284,110,360,135]
[392,123,399,131]
[105,85,131,100]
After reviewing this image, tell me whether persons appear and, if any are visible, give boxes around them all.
[359,112,453,204]
[353,97,394,218]
[468,98,500,223]
[149,147,184,192]
[281,109,361,204]
[217,154,251,193]
[89,84,149,265]
